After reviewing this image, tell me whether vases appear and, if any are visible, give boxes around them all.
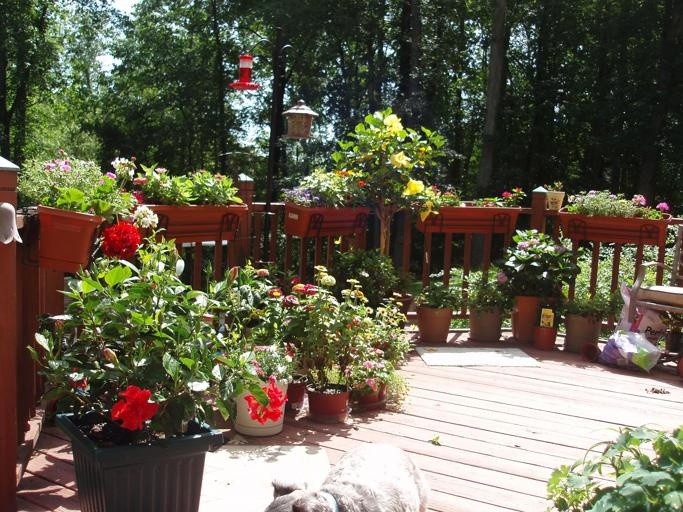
[134,204,248,242]
[417,207,521,234]
[282,203,371,239]
[306,384,348,425]
[351,379,388,410]
[285,382,305,410]
[233,379,288,438]
[53,414,223,512]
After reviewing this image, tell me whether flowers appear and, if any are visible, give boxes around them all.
[495,232,574,289]
[281,172,364,207]
[432,184,525,207]
[29,152,117,215]
[133,167,225,204]
[227,264,412,376]
[43,222,227,433]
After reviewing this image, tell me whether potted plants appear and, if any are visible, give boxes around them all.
[461,273,510,344]
[562,289,612,355]
[533,301,559,350]
[545,180,566,211]
[37,204,102,275]
[415,273,462,344]
[512,296,551,344]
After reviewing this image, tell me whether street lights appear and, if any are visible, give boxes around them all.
[229,36,322,244]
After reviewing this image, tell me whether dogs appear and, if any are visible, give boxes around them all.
[264,444,428,512]
